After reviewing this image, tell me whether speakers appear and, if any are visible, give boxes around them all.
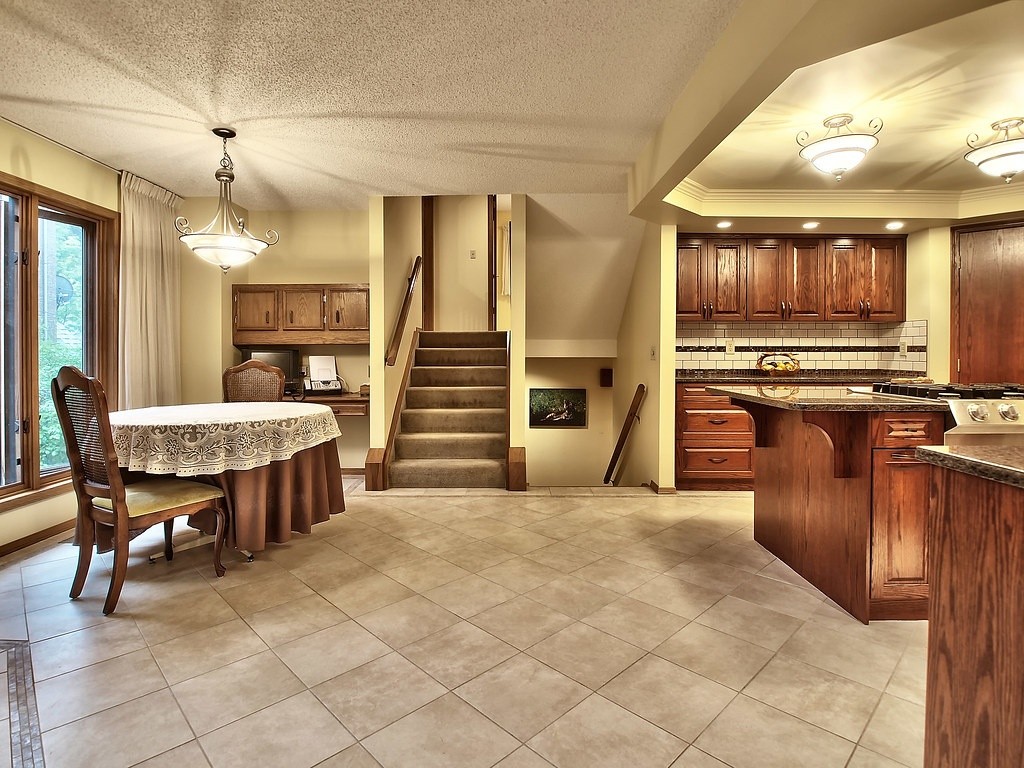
[600,369,613,387]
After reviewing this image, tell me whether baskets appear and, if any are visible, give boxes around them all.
[754,352,800,377]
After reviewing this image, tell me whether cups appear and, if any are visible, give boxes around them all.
[872,381,1024,399]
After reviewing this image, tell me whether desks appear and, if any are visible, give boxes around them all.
[70,402,346,562]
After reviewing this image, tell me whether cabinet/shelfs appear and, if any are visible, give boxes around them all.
[230,282,370,417]
[676,233,746,322]
[746,233,826,322]
[750,404,958,626]
[825,233,909,322]
[675,382,757,491]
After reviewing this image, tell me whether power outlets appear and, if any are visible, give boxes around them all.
[725,340,736,354]
[899,341,907,356]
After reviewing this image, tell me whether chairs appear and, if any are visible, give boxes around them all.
[50,365,228,615]
[221,358,287,403]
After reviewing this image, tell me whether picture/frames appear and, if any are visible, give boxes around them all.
[528,387,589,430]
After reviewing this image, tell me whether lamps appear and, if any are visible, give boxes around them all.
[795,113,884,184]
[175,127,280,275]
[962,115,1024,184]
